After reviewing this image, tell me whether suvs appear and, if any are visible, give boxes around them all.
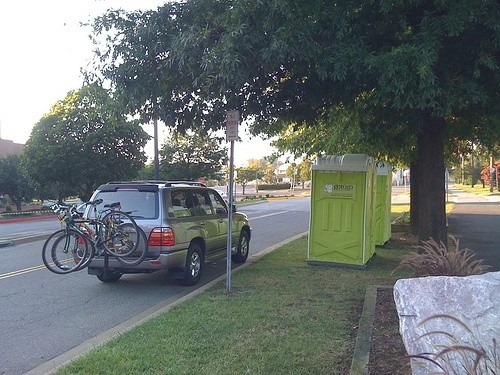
[74,179,252,286]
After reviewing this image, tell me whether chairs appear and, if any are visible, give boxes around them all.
[185,195,209,216]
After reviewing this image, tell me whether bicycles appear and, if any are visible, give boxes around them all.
[42,198,147,273]
